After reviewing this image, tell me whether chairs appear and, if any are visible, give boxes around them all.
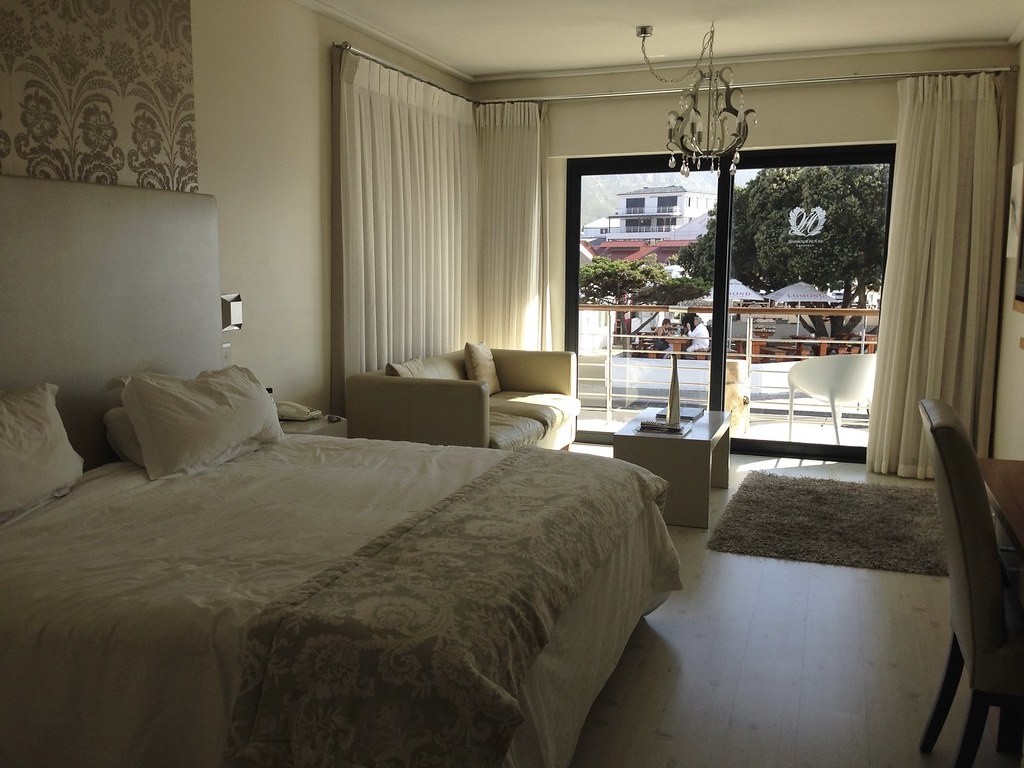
[787,354,877,444]
[914,398,1024,768]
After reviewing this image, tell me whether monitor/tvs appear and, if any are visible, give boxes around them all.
[1012,207,1024,313]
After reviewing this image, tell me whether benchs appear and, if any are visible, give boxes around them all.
[630,338,861,364]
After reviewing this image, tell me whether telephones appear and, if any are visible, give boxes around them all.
[277,401,322,421]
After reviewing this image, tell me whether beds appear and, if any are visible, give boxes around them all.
[0,175,684,765]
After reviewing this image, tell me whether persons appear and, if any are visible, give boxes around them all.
[654,313,710,359]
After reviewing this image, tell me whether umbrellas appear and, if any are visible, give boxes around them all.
[764,281,837,346]
[701,278,763,350]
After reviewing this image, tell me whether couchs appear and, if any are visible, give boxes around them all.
[344,349,582,453]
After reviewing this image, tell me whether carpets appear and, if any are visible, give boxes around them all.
[709,470,949,577]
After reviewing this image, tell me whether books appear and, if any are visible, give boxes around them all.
[637,405,705,436]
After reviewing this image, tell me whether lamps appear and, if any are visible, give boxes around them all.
[635,21,757,178]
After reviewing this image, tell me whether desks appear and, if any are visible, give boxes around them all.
[791,335,812,358]
[978,457,1024,575]
[613,406,734,529]
[734,339,768,364]
[814,336,833,356]
[665,338,689,359]
[857,333,878,355]
[278,413,348,438]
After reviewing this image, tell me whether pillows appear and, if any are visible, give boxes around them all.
[0,382,84,522]
[120,365,285,481]
[465,340,500,397]
